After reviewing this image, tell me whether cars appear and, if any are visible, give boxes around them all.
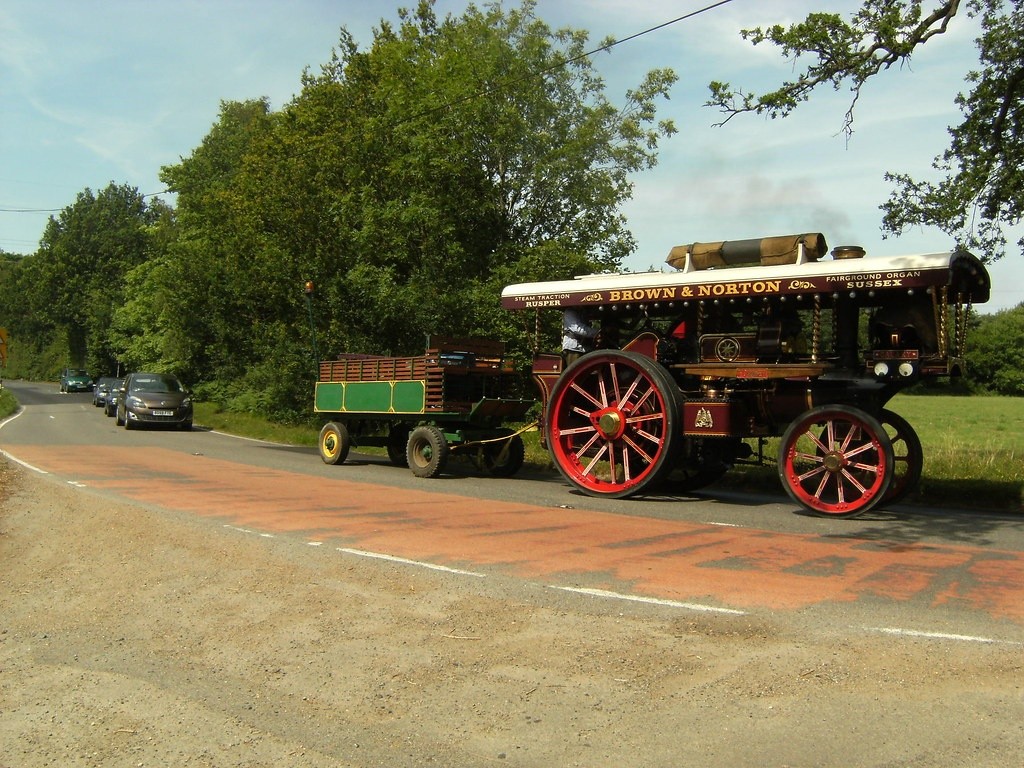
[116,372,194,431]
[59,368,94,393]
[92,377,117,407]
[102,379,123,417]
